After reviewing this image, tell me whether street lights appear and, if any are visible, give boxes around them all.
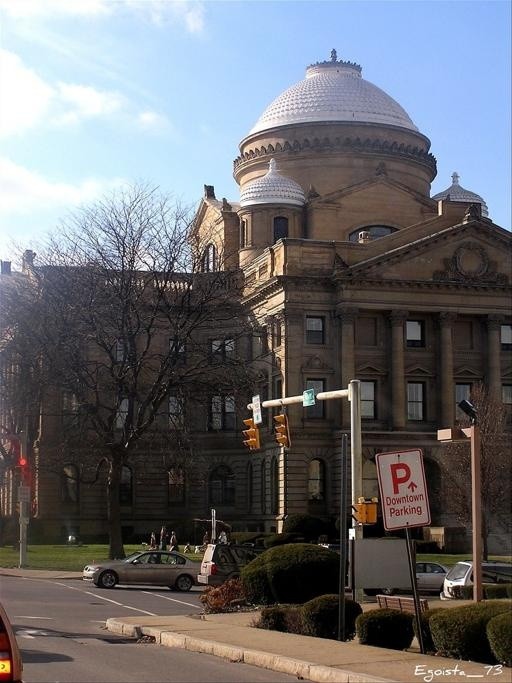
[436,397,485,604]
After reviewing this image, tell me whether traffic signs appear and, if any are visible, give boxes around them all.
[252,395,263,423]
[303,387,315,406]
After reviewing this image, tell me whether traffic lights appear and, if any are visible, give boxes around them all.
[241,415,261,452]
[273,411,292,451]
[15,465,24,482]
[18,456,28,465]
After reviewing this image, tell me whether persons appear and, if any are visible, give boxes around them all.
[149,525,228,555]
[151,554,161,564]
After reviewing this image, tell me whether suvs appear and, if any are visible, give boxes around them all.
[197,543,268,586]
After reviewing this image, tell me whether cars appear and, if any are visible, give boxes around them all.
[82,549,204,590]
[367,559,512,601]
[0,601,24,680]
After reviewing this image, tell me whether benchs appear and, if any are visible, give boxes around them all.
[376,594,428,614]
